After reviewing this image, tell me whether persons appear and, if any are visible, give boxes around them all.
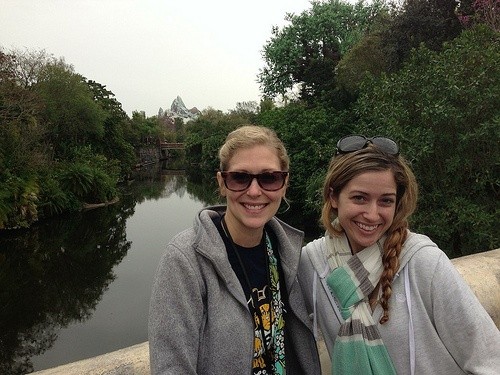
[148,124,321,375]
[296,134,500,375]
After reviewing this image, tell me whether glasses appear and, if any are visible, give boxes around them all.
[220,169,288,192]
[334,134,400,159]
